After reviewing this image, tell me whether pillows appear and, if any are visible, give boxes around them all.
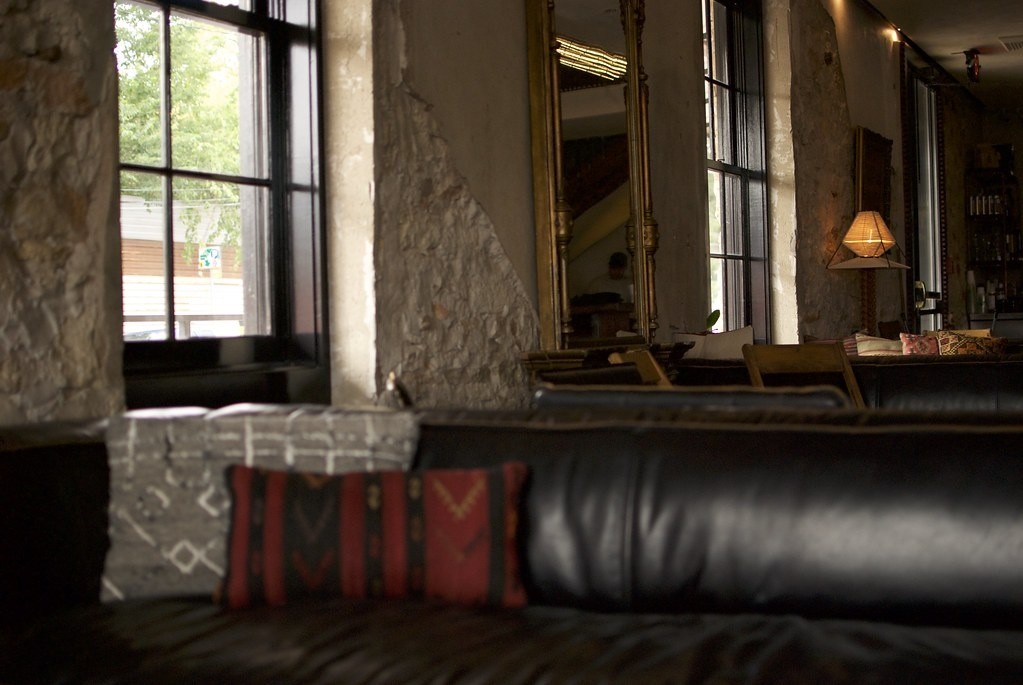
[818,328,868,356]
[856,334,903,356]
[899,333,939,355]
[214,460,526,609]
[99,402,418,603]
[937,330,1008,359]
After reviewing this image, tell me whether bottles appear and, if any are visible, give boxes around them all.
[967,230,1022,316]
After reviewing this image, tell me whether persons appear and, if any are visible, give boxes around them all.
[590,252,635,296]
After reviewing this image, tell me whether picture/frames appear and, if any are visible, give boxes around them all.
[855,126,893,226]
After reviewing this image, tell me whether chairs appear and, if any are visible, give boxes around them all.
[743,342,865,410]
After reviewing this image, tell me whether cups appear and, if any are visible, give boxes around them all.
[968,194,1008,215]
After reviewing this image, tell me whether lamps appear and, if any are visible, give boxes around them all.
[826,211,913,270]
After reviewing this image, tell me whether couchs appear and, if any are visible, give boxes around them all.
[1,413,1023,685]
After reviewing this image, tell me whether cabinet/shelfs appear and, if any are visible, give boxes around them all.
[965,143,1023,313]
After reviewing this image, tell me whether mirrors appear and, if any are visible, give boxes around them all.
[554,0,648,351]
